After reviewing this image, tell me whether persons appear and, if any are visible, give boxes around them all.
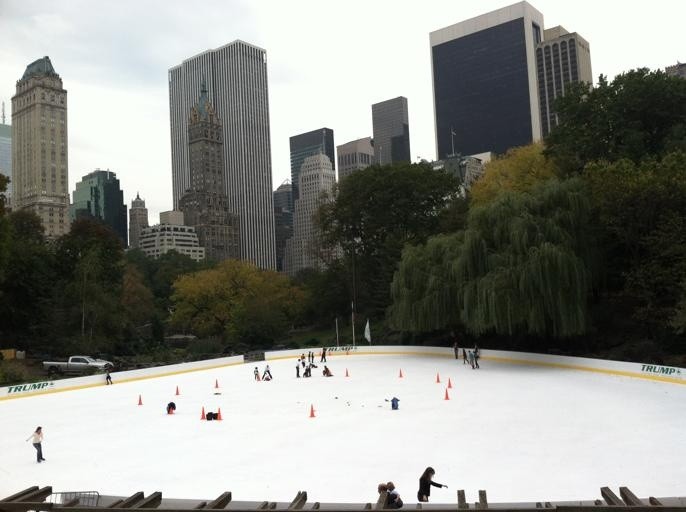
[454,342,481,369]
[378,484,403,509]
[294,347,333,378]
[385,481,400,502]
[254,366,260,379]
[104,367,113,384]
[417,466,449,502]
[26,426,45,463]
[262,364,272,379]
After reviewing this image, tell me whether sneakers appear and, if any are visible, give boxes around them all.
[37,457,45,462]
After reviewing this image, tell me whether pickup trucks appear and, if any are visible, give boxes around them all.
[42,356,115,380]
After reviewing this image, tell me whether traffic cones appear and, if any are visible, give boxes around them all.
[169,407,174,414]
[400,369,403,377]
[217,408,223,421]
[346,369,348,377]
[138,395,143,405]
[445,389,449,400]
[201,406,206,419]
[436,372,441,383]
[176,386,180,395]
[311,405,315,417]
[448,378,452,389]
[216,380,219,388]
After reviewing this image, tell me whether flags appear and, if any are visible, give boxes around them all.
[364,320,372,343]
